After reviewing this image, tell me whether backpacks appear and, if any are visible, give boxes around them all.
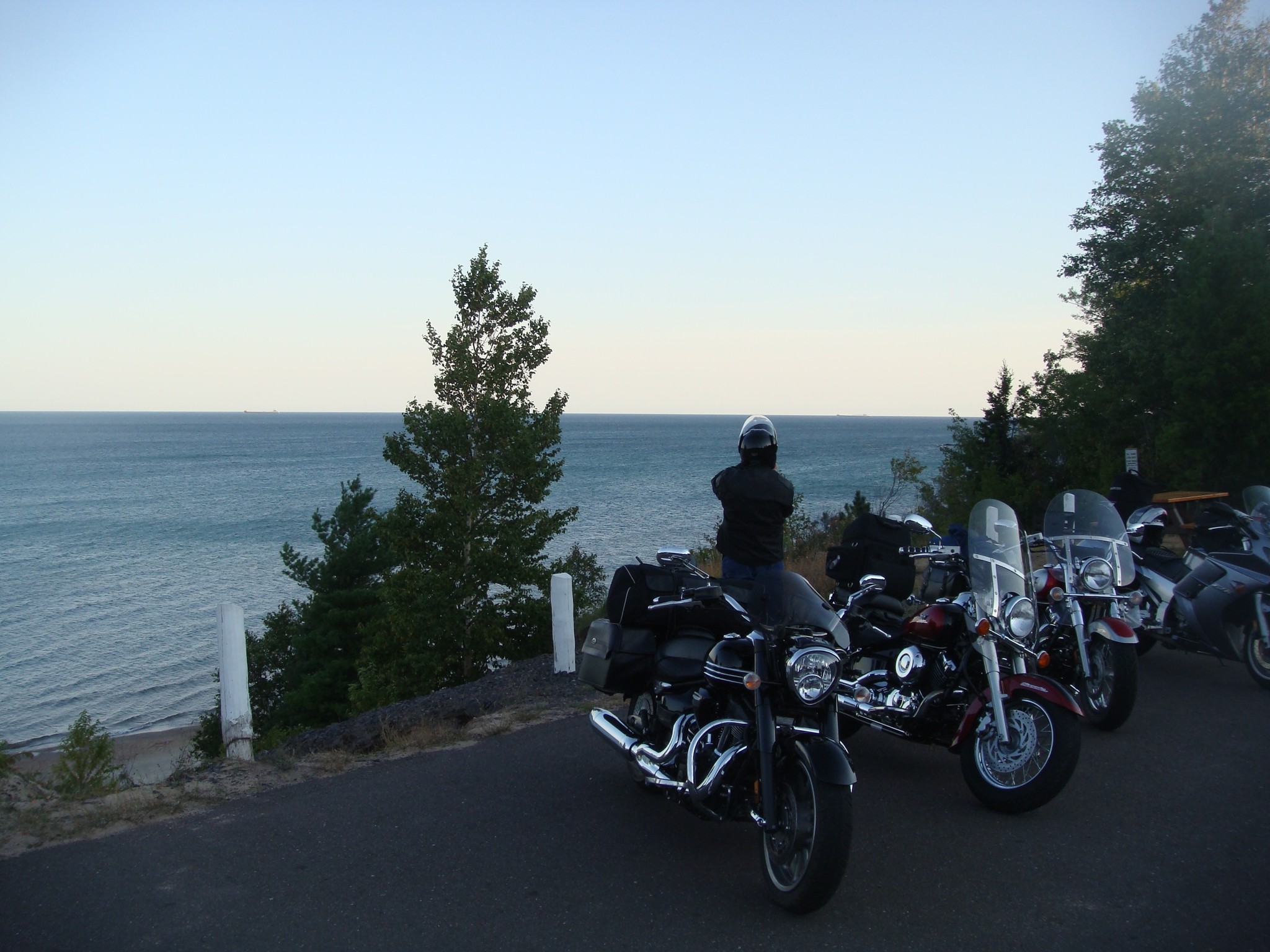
[1109,469,1154,524]
[918,523,1009,600]
[825,512,916,602]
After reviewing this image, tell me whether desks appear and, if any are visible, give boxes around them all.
[1151,490,1230,551]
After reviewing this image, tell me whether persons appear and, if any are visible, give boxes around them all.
[709,415,794,582]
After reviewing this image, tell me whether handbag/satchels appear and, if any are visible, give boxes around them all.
[606,557,767,632]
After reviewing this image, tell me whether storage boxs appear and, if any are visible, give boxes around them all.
[920,562,971,602]
[578,619,655,695]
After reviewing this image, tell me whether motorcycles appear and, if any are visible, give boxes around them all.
[923,485,1270,731]
[825,499,1085,812]
[578,546,883,912]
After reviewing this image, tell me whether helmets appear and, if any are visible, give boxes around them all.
[739,414,778,471]
[1126,505,1167,547]
